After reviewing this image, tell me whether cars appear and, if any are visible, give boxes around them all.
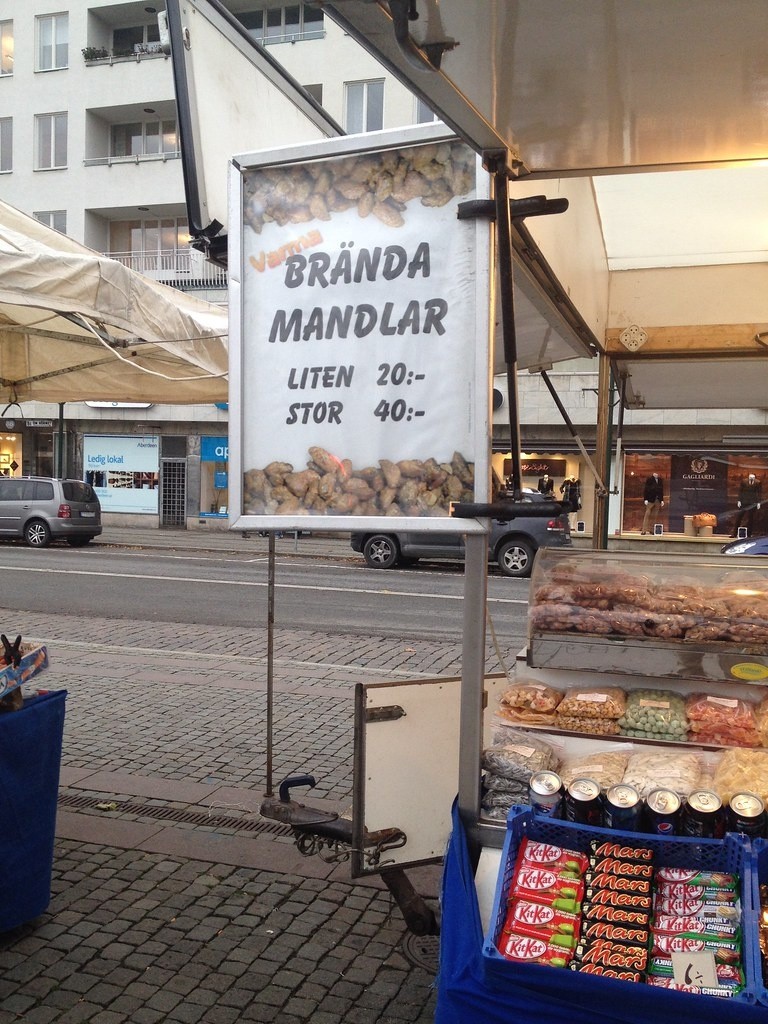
[720,535,768,557]
[348,488,573,578]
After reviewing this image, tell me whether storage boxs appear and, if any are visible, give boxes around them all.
[480,803,768,1011]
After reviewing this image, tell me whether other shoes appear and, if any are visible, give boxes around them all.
[650,530,654,535]
[729,535,737,538]
[641,531,646,535]
[747,534,751,538]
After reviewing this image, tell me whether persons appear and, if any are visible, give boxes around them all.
[538,475,554,496]
[729,474,762,538]
[641,472,664,535]
[506,473,514,490]
[559,475,583,531]
[241,529,283,540]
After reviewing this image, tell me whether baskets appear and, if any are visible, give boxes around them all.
[481,804,768,1009]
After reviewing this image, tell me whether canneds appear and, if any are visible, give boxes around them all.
[529,769,768,840]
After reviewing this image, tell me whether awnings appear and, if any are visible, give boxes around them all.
[0,198,230,404]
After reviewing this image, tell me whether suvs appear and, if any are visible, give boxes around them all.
[0,476,102,548]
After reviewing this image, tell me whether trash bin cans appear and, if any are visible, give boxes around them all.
[0,632,69,934]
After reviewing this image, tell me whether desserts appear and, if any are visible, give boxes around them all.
[701,873,738,965]
